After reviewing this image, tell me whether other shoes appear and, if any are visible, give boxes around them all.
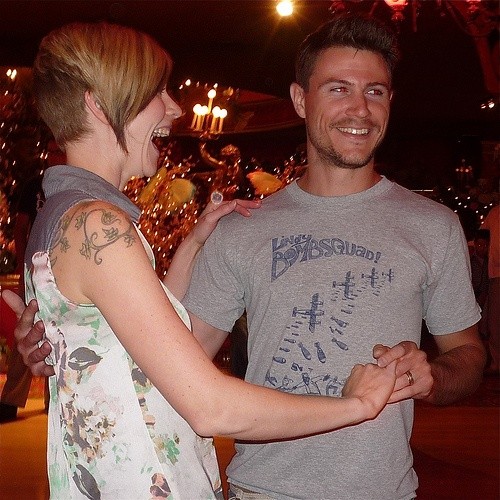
[483,367,499,376]
[0,402,17,423]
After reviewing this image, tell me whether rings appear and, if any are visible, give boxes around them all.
[406,371,414,386]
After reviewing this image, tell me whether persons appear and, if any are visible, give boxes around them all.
[0,350,33,424]
[1,23,486,499]
[22,21,400,500]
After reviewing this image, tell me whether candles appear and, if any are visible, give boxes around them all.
[191,88,228,133]
